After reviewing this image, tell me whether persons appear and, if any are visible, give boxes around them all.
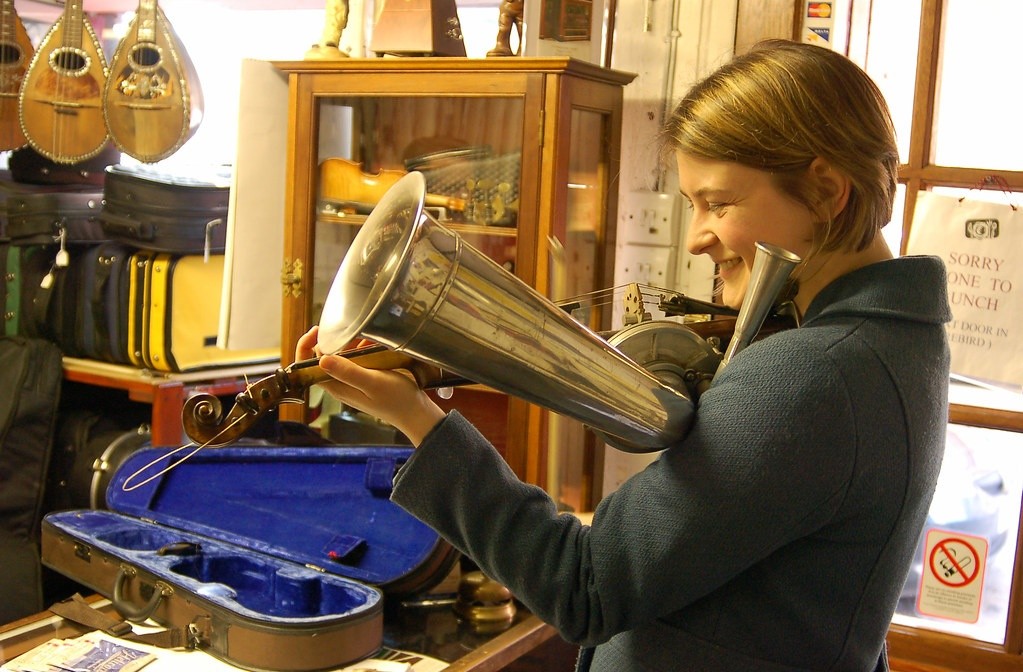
[295,40,953,672]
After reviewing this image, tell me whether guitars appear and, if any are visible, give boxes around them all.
[103,0,204,166]
[0,0,111,164]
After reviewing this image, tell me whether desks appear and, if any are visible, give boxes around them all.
[0,557,580,672]
[63,359,279,448]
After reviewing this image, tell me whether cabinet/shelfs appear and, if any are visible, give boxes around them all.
[268,54,639,521]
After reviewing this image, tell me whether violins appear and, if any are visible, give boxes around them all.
[317,158,522,228]
[182,280,797,448]
